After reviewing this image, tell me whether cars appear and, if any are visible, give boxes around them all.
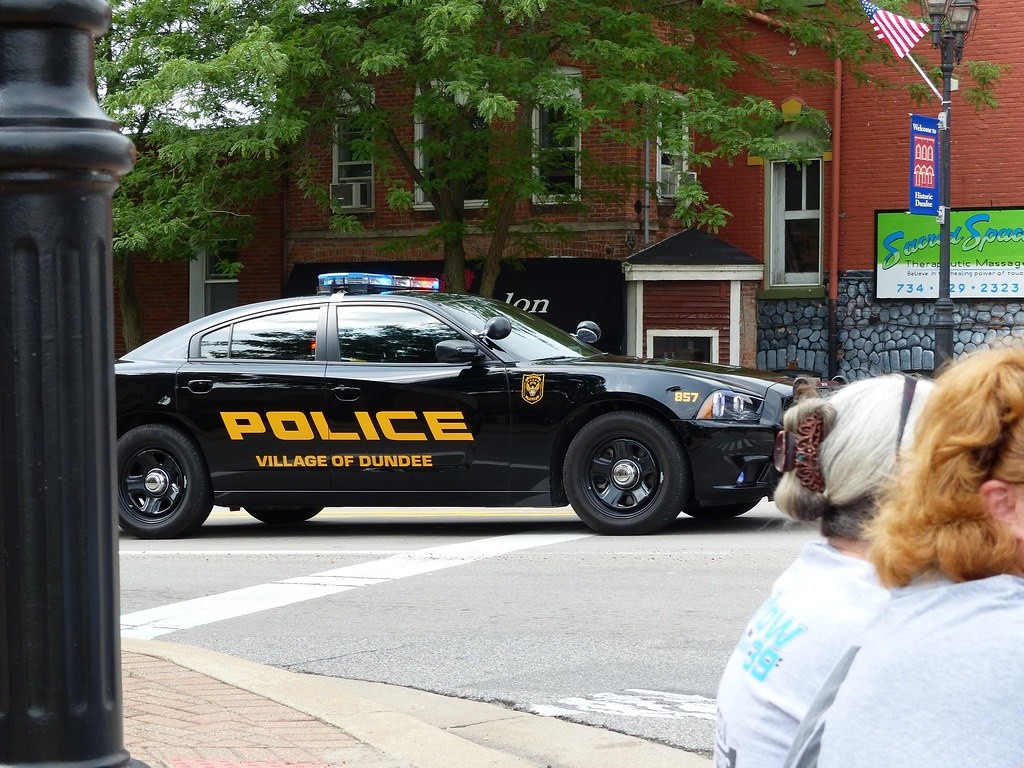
[115,272,795,540]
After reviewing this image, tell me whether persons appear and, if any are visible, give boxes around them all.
[712,345,1023,767]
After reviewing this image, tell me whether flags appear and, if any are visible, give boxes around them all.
[863,1,930,61]
